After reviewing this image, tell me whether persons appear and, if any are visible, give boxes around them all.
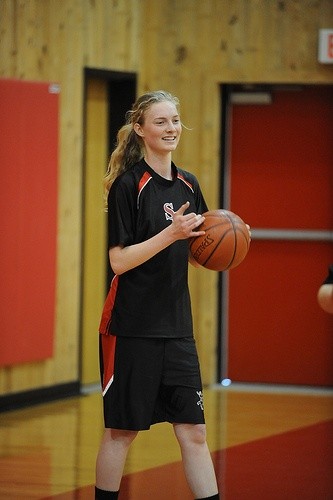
[94,91,252,500]
[317,263,333,315]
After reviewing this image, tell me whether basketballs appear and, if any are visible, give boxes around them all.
[189,210,250,271]
